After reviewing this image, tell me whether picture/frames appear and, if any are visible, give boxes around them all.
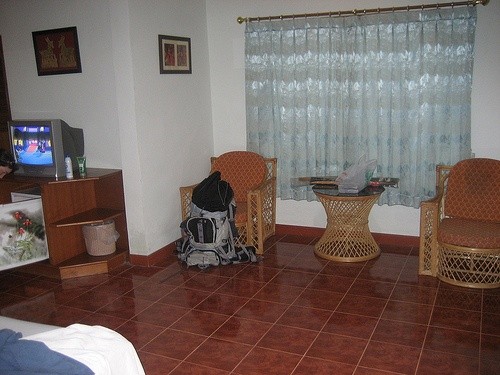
[31,26,83,77]
[158,34,193,75]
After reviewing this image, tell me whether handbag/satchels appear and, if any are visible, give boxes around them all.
[335,153,378,185]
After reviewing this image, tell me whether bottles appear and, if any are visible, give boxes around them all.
[377,168,383,182]
[64,152,74,180]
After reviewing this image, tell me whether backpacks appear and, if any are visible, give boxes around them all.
[174,170,258,268]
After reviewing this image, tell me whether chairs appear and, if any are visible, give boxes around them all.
[415,155,500,289]
[176,150,279,255]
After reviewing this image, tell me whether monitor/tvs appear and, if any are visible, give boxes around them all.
[8,119,84,178]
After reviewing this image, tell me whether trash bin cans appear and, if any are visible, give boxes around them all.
[82,220,117,256]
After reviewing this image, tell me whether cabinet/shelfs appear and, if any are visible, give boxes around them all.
[0,167,128,281]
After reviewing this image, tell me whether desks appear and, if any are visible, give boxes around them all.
[313,183,384,263]
